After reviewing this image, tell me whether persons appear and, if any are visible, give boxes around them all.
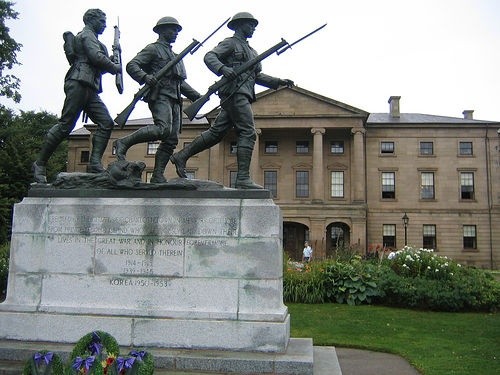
[169,12,295,188]
[387,248,395,259]
[112,17,205,183]
[302,241,313,263]
[31,8,122,183]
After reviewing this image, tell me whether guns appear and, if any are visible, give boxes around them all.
[182,23,328,121]
[112,15,124,95]
[114,17,232,130]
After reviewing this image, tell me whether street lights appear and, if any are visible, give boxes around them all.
[402,212,410,246]
